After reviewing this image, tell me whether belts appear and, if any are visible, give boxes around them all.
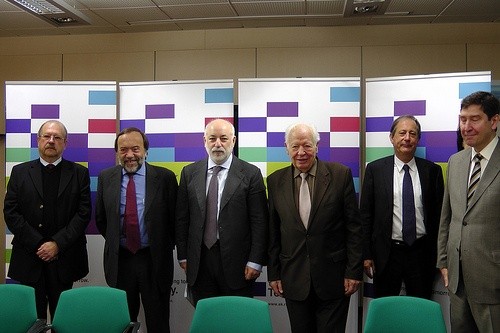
[392,240,408,246]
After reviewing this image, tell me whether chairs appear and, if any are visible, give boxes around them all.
[363,296,446,333]
[36,286,141,333]
[189,296,273,333]
[0,283,48,333]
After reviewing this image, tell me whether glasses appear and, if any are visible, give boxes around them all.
[39,134,64,140]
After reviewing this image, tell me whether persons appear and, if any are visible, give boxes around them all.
[266,122,363,332]
[360,115,445,298]
[94,128,178,331]
[177,118,267,305]
[2,119,93,333]
[436,90,500,332]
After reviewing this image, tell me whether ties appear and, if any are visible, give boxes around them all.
[402,165,416,246]
[47,164,54,169]
[204,166,223,249]
[123,173,141,254]
[467,154,484,209]
[299,172,311,230]
